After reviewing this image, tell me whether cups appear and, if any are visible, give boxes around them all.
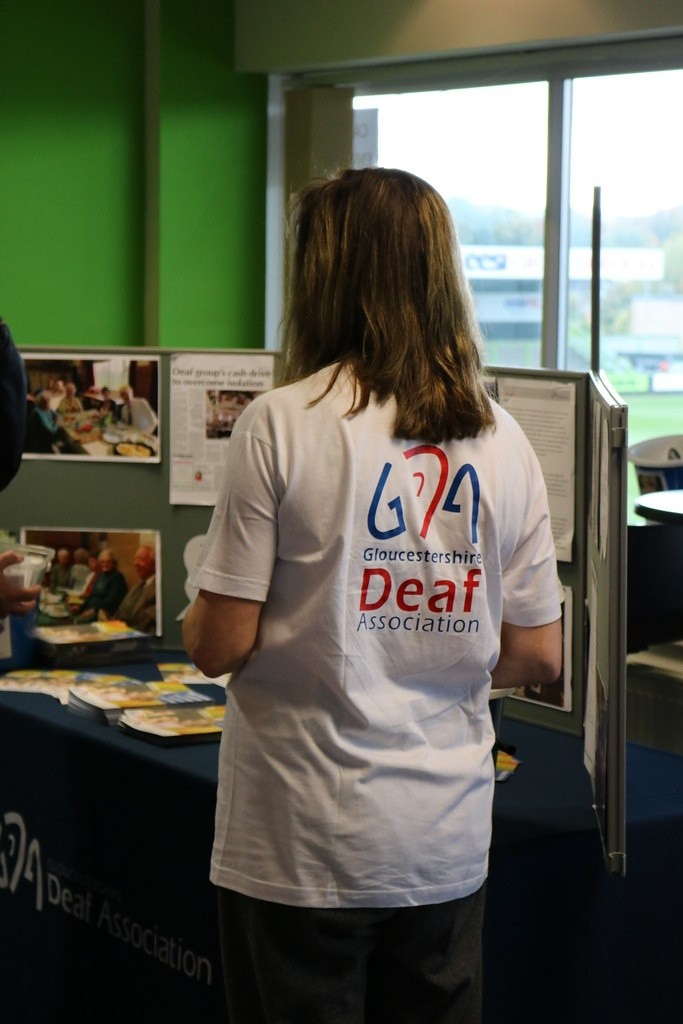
[0,544,55,605]
[628,435,683,496]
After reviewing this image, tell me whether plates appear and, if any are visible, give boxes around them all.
[130,433,157,452]
[634,490,683,519]
[102,432,122,444]
[114,442,154,458]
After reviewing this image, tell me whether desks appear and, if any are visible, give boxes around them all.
[0,646,682,1024]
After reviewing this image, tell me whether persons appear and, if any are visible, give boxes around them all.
[180,168,566,1024]
[0,555,41,617]
[47,546,157,634]
[27,377,158,453]
[205,390,253,439]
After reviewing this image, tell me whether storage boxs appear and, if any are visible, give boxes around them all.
[32,618,152,663]
